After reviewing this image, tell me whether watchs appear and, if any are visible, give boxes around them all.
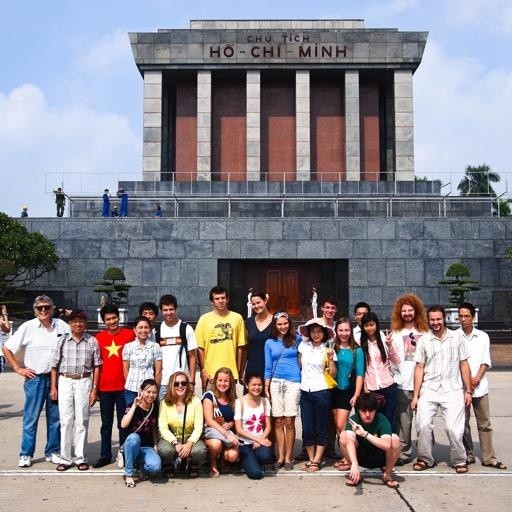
[189,381,195,385]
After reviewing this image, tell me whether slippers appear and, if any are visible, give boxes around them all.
[382,477,399,488]
[346,478,363,486]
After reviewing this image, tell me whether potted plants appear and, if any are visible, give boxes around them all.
[438,263,479,330]
[93,267,132,332]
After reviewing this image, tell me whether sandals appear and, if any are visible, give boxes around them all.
[78,463,89,470]
[310,462,321,470]
[56,464,72,471]
[413,462,428,471]
[466,453,475,466]
[334,460,343,468]
[339,463,348,471]
[456,465,468,473]
[482,461,506,469]
[305,460,312,468]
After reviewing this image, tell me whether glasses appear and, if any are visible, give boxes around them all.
[35,305,51,311]
[174,381,187,386]
[409,333,416,346]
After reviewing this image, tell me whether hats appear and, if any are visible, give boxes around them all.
[69,309,87,320]
[298,318,334,339]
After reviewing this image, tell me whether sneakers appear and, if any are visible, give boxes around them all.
[189,467,199,478]
[45,453,60,464]
[126,476,136,487]
[140,472,149,480]
[165,465,174,478]
[395,458,412,465]
[18,456,32,467]
[272,461,283,469]
[284,463,293,470]
[92,458,111,468]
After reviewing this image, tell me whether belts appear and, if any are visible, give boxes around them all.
[61,373,90,379]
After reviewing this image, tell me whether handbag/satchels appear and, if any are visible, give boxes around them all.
[388,364,407,385]
[204,417,238,448]
[323,348,338,390]
[117,448,124,468]
[180,455,190,473]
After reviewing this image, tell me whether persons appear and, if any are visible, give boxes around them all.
[111,206,118,217]
[390,294,434,466]
[157,371,207,478]
[116,188,128,217]
[352,302,386,346]
[122,315,163,408]
[455,303,507,469]
[247,288,253,318]
[103,189,110,217]
[410,305,473,474]
[152,206,162,217]
[0,305,10,373]
[311,287,318,317]
[20,204,29,217]
[234,373,273,480]
[329,316,365,471]
[356,312,401,434]
[338,393,401,487]
[195,286,247,394]
[53,188,65,217]
[264,311,302,471]
[49,309,104,471]
[2,295,69,467]
[139,302,160,345]
[200,367,241,477]
[321,297,337,330]
[155,294,199,401]
[92,304,136,468]
[121,379,163,488]
[298,318,338,472]
[244,291,276,398]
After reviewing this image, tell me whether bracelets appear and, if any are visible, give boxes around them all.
[92,384,97,389]
[223,430,231,439]
[467,392,472,395]
[172,440,178,447]
[263,384,270,386]
[364,431,370,438]
